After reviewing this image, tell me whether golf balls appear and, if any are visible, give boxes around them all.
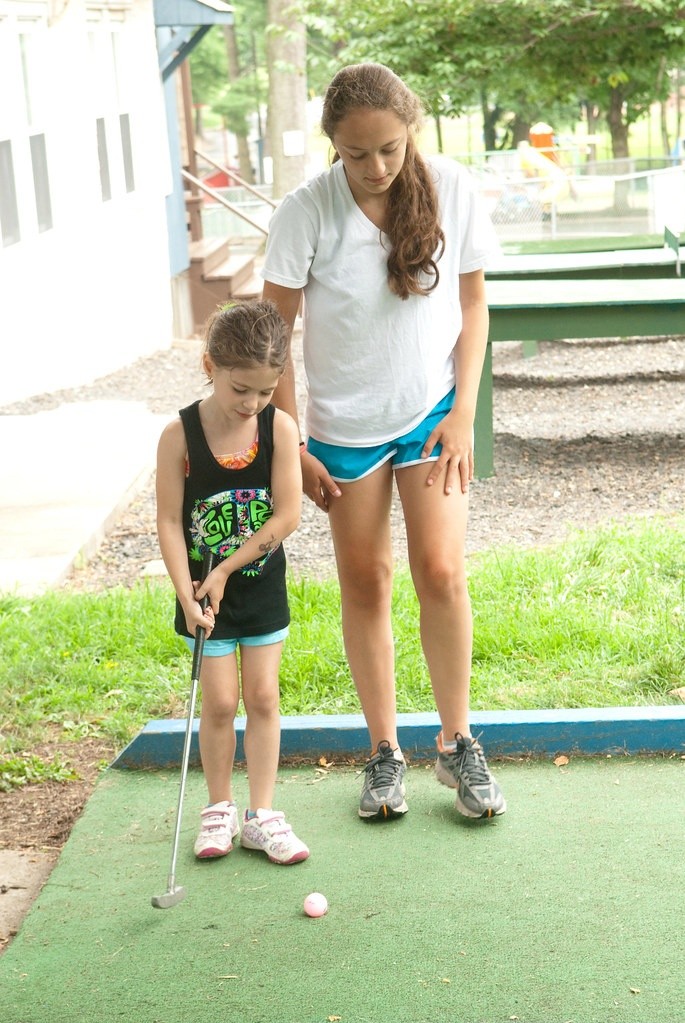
[304,892,328,918]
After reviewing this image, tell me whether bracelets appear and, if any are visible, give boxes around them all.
[299,442,307,456]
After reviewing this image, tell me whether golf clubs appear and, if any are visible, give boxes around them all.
[151,550,216,910]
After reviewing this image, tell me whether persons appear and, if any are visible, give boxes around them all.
[156,299,310,866]
[260,61,506,823]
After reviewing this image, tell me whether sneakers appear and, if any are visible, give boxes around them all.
[355,740,408,818]
[193,801,241,858]
[240,808,309,865]
[436,731,507,818]
